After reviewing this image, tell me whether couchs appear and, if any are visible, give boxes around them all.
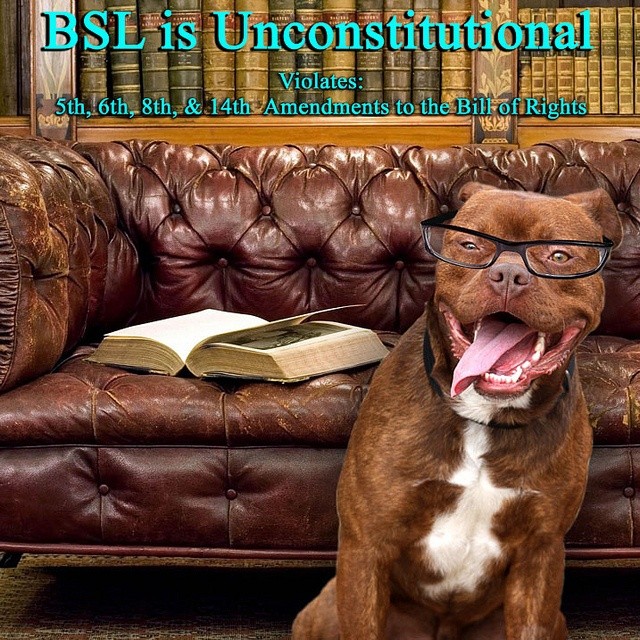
[2,135,640,569]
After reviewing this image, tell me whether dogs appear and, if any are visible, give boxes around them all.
[292,181,624,640]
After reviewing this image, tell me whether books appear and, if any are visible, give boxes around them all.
[83,303,391,386]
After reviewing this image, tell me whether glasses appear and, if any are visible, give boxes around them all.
[421,211,613,279]
